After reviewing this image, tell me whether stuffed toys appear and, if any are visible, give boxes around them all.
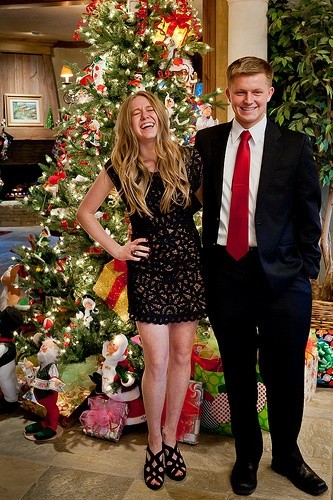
[25,337,61,439]
[102,333,147,426]
[0,264,34,411]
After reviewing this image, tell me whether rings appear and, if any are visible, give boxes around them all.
[132,250,136,255]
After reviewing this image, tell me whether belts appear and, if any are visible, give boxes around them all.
[216,244,259,258]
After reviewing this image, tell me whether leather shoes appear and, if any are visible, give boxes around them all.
[230,455,261,496]
[271,458,328,495]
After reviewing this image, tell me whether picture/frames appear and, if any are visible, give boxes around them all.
[3,93,44,127]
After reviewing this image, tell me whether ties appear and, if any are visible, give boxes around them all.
[226,130,252,262]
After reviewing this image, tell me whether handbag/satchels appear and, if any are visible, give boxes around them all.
[190,344,319,438]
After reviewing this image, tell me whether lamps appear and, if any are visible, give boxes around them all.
[60,65,73,88]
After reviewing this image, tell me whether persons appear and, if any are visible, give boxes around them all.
[78,92,208,490]
[190,55,327,495]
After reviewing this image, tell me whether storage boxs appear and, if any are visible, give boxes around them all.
[161,381,203,445]
[19,376,96,429]
[92,258,129,322]
[81,399,131,442]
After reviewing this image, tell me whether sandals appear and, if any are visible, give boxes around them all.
[162,441,187,481]
[144,445,164,490]
